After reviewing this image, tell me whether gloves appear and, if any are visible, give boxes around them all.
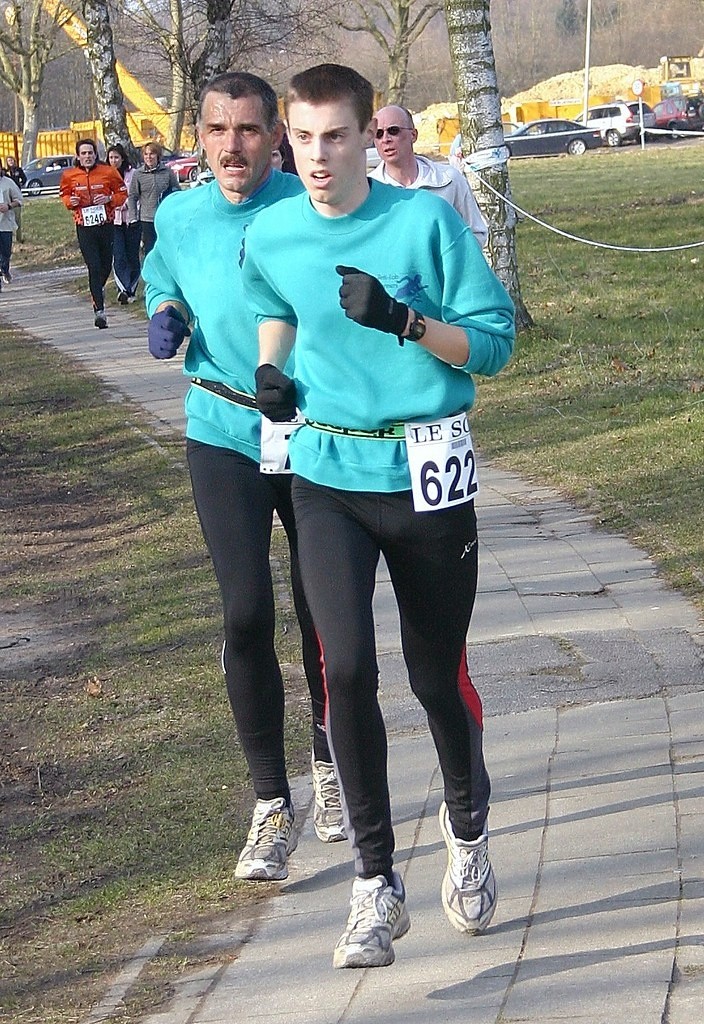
[147,305,192,360]
[336,265,409,336]
[254,363,296,422]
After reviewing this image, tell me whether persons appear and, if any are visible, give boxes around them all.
[61,139,181,329]
[239,64,518,969]
[448,134,463,173]
[272,143,288,175]
[368,105,489,247]
[0,156,29,291]
[141,72,352,881]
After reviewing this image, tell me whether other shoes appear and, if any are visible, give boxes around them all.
[0,269,12,283]
[93,310,107,329]
[117,291,137,305]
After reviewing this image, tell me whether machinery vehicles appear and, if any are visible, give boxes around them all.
[657,56,704,100]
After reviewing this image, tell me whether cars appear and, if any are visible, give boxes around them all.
[133,146,209,182]
[504,119,603,156]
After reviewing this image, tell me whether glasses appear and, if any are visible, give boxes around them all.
[376,125,415,139]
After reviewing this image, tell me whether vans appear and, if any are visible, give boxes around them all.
[19,155,75,196]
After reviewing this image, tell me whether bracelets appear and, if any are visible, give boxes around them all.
[8,204,11,210]
[109,196,113,201]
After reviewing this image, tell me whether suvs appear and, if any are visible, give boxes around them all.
[573,100,657,147]
[652,96,704,138]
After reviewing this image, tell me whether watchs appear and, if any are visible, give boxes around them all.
[398,309,426,347]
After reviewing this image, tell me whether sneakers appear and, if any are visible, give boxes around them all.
[438,801,498,935]
[235,797,298,880]
[332,870,410,969]
[311,744,348,843]
